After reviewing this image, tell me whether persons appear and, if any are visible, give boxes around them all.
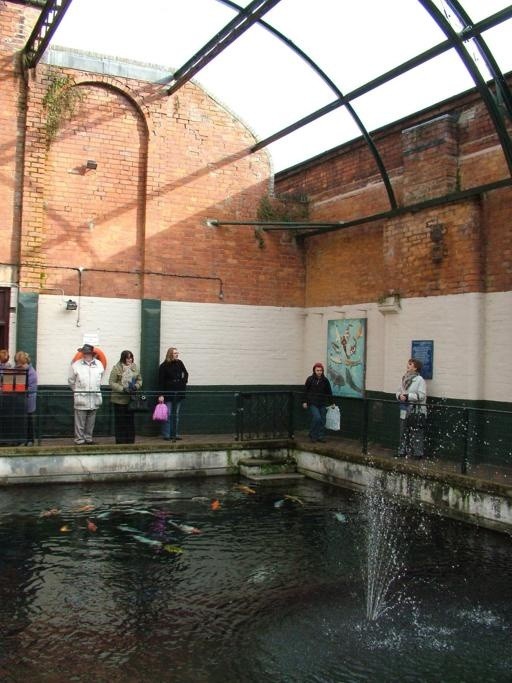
[157,347,189,437]
[0,349,11,386]
[69,343,105,445]
[302,362,335,443]
[393,356,429,459]
[109,350,143,443]
[14,350,39,446]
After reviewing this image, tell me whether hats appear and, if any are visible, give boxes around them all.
[77,343,97,356]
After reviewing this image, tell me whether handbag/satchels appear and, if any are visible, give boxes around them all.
[406,391,427,429]
[324,403,341,432]
[127,383,154,412]
[152,397,168,422]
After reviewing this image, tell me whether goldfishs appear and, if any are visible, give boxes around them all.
[284,493,305,506]
[85,518,99,533]
[210,497,221,511]
[273,499,285,509]
[38,507,59,519]
[129,531,162,549]
[232,485,256,495]
[168,518,202,534]
[61,522,74,533]
[82,503,95,512]
[332,510,349,526]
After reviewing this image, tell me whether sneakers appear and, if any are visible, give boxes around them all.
[162,435,183,441]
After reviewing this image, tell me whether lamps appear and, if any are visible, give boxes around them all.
[62,299,79,314]
[83,161,98,174]
